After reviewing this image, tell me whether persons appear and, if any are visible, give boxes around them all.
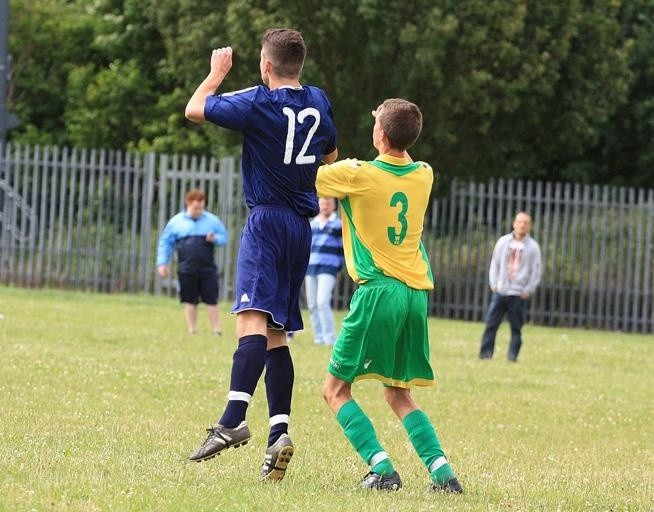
[185,28,338,484]
[312,98,462,493]
[479,211,541,361]
[304,194,346,346]
[158,189,228,338]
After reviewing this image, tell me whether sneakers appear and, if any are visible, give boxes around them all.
[427,477,463,493]
[188,420,250,463]
[259,433,294,482]
[357,472,402,491]
